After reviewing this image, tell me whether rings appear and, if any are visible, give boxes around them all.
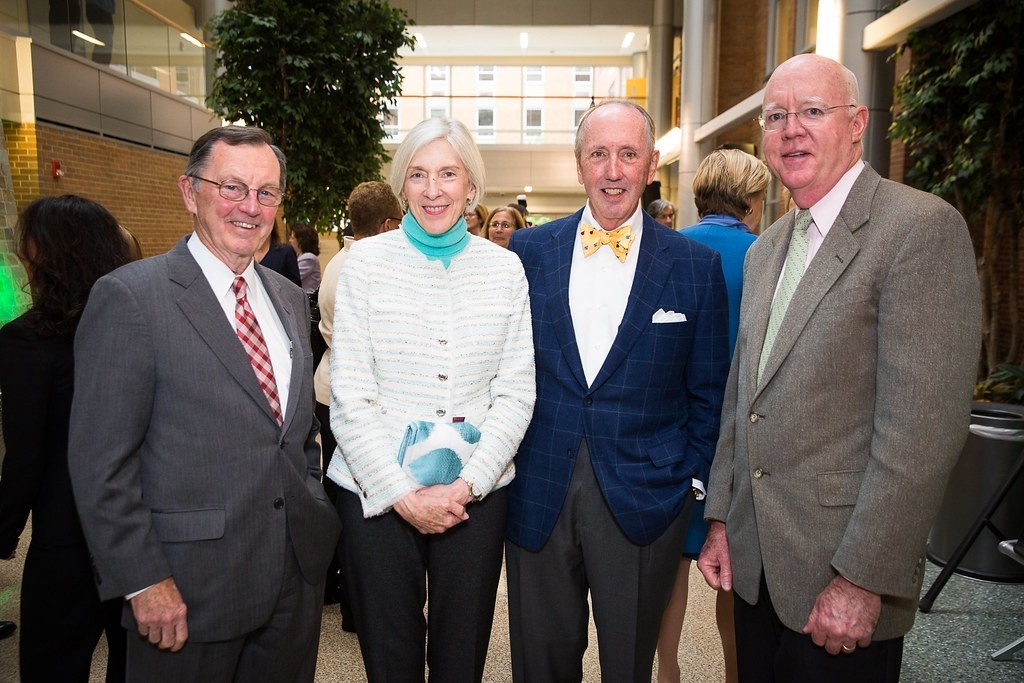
[843,644,856,651]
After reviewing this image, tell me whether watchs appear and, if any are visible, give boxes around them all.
[469,485,482,502]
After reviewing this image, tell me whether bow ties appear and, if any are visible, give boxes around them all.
[581,224,632,263]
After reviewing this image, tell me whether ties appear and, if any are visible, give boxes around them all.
[755,208,814,386]
[231,277,282,428]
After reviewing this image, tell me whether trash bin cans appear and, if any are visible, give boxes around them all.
[923,402,1024,585]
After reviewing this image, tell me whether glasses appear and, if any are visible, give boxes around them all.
[658,214,672,219]
[463,212,478,218]
[192,176,285,208]
[753,104,857,133]
[381,217,400,224]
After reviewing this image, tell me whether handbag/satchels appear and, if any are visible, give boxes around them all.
[397,420,481,487]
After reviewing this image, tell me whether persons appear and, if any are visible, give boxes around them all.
[655,150,775,683]
[66,126,341,683]
[325,119,536,683]
[254,181,527,631]
[781,185,798,216]
[647,198,677,228]
[695,55,983,683]
[0,194,144,683]
[508,101,731,683]
